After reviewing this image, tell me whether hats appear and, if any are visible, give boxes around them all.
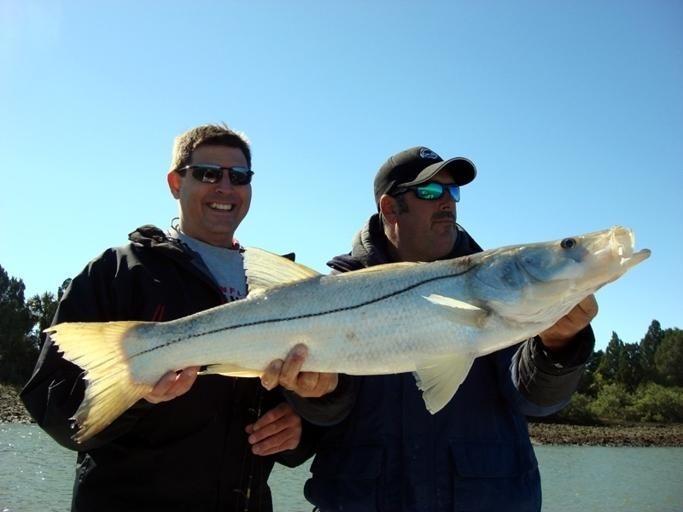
[371,145,477,214]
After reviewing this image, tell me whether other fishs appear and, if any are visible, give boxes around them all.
[42,225,652,444]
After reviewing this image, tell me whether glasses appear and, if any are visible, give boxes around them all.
[388,181,461,203]
[175,163,255,187]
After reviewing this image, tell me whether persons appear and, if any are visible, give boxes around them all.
[260,146,599,512]
[17,124,303,512]
